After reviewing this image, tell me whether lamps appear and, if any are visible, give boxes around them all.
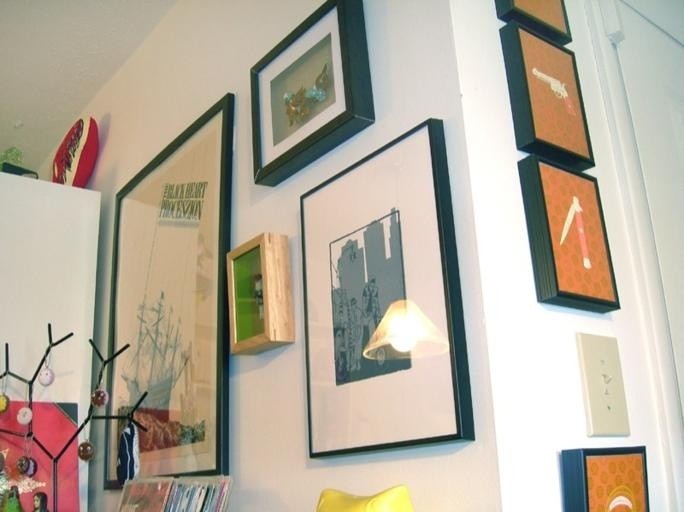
[362,299,450,360]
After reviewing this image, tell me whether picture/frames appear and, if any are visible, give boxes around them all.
[518,154,621,314]
[562,445,649,512]
[226,231,295,356]
[499,20,595,172]
[102,93,232,490]
[299,118,476,459]
[250,0,374,187]
[494,0,572,46]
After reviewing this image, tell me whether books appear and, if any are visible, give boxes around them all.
[115,475,232,512]
